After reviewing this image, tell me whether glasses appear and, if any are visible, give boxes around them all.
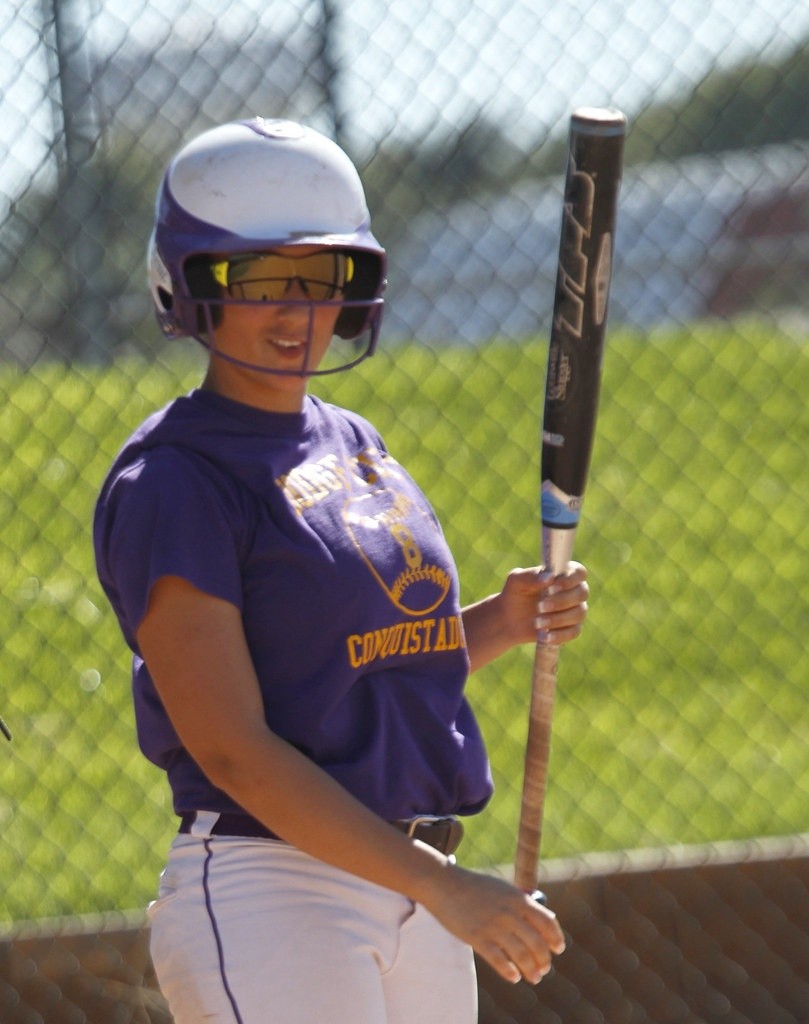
[205,250,354,303]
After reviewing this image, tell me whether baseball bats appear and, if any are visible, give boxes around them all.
[512,107,630,903]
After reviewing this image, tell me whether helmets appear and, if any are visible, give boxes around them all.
[145,118,388,341]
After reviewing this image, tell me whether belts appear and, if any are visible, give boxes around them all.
[177,812,467,857]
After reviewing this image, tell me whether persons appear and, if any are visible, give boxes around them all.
[93,119,589,1024]
